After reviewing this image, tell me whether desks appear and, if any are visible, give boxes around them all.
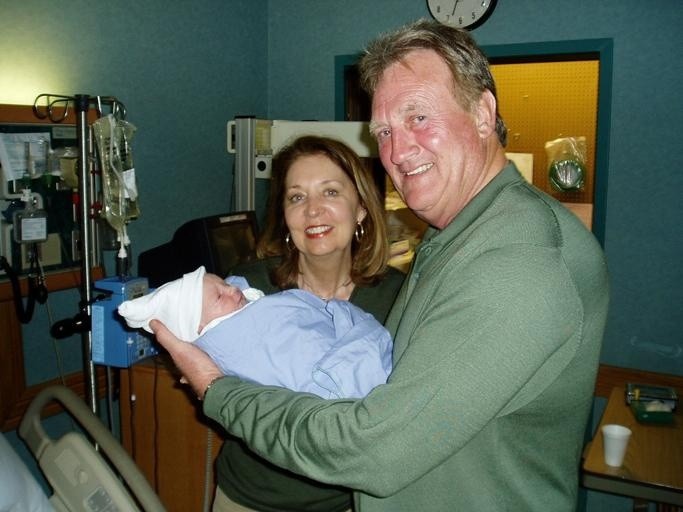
[117,355,230,512]
[582,385,682,512]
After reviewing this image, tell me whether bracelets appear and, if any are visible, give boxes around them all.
[204,373,225,398]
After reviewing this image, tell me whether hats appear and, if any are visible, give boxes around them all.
[117,265,206,340]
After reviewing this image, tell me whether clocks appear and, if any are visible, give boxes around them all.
[426,0,497,31]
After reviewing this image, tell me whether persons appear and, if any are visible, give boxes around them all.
[147,16,609,512]
[119,265,393,401]
[178,136,406,512]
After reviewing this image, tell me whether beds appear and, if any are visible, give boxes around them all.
[0,383,167,512]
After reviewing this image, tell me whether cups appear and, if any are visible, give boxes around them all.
[601,423,632,469]
